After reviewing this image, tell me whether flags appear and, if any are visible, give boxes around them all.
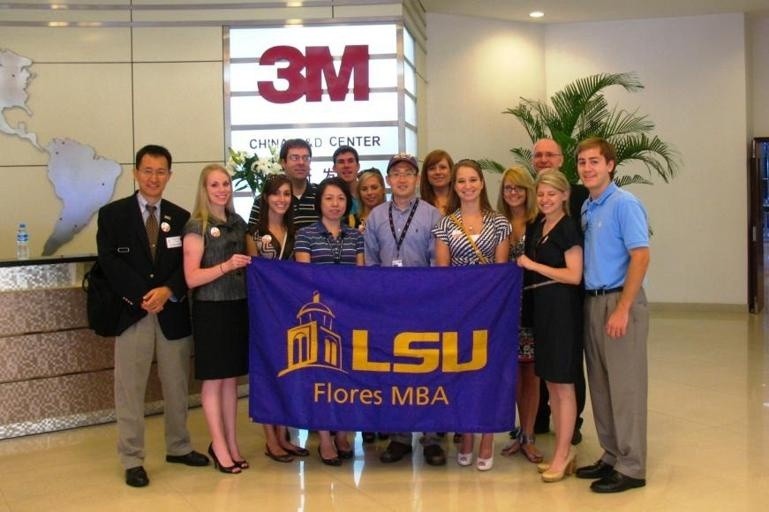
[246,258,520,434]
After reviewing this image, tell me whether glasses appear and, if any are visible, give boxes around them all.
[290,155,311,161]
[535,154,562,158]
[503,187,525,193]
[390,172,416,178]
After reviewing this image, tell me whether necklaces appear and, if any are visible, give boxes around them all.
[462,217,481,233]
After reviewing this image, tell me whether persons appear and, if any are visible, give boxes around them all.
[497,164,544,462]
[363,154,449,466]
[343,169,390,443]
[181,166,258,474]
[82,145,210,486]
[248,139,322,239]
[295,178,365,467]
[518,168,584,483]
[432,159,513,472]
[334,146,364,219]
[245,175,310,462]
[509,139,591,445]
[421,150,467,443]
[575,139,649,493]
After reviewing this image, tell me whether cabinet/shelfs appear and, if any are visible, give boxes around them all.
[0,255,248,441]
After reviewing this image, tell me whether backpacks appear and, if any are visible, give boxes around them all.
[83,200,144,337]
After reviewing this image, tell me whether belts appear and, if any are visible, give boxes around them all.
[584,287,624,295]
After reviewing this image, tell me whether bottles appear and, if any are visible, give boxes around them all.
[16,224,30,260]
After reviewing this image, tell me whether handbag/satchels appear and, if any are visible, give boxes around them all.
[82,262,119,338]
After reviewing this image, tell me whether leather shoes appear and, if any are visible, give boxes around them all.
[280,441,309,456]
[167,450,209,467]
[521,443,543,463]
[591,470,645,492]
[576,460,613,478]
[126,466,149,486]
[501,437,521,456]
[380,441,413,463]
[423,445,447,465]
[265,443,294,462]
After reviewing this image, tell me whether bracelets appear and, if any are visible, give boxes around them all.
[219,263,225,276]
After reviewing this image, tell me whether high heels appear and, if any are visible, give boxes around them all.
[334,438,353,459]
[542,445,578,481]
[537,459,572,475]
[208,441,241,473]
[458,451,472,466]
[318,444,343,466]
[476,440,495,471]
[232,458,248,468]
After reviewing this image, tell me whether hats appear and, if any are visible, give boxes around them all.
[387,153,418,173]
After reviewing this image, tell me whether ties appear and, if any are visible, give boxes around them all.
[145,205,159,262]
